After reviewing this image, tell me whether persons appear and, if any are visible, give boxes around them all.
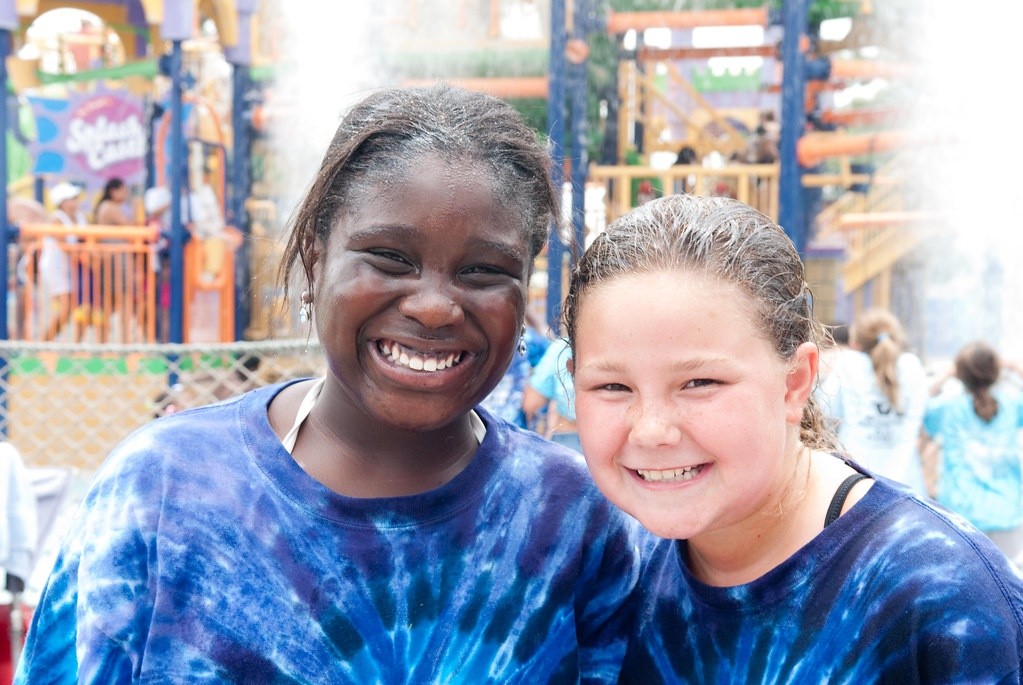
[808,309,1023,561]
[565,192,1023,685]
[8,86,661,685]
[478,301,586,461]
[37,178,204,343]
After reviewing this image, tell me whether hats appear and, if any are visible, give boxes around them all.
[49,182,80,207]
